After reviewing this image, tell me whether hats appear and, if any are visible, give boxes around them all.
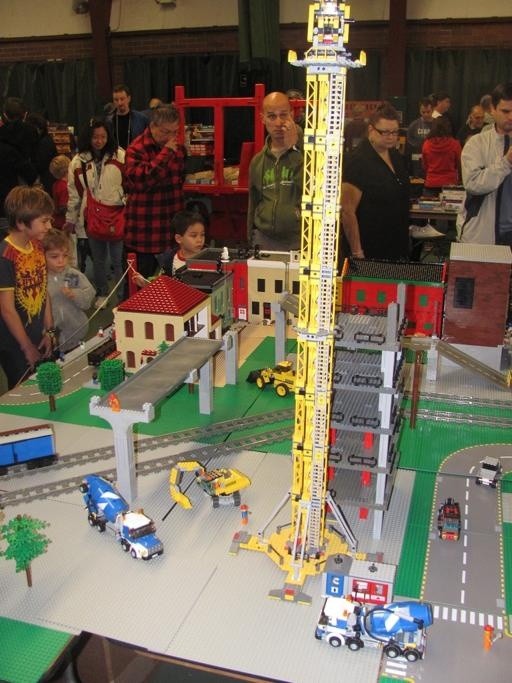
[103,103,117,114]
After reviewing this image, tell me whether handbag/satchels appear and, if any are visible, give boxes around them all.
[84,187,127,242]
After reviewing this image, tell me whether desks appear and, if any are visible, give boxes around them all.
[407,207,457,262]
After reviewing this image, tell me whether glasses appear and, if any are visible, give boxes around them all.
[369,123,400,136]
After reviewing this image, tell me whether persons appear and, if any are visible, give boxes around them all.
[250,91,305,262]
[340,82,512,264]
[0,82,213,397]
